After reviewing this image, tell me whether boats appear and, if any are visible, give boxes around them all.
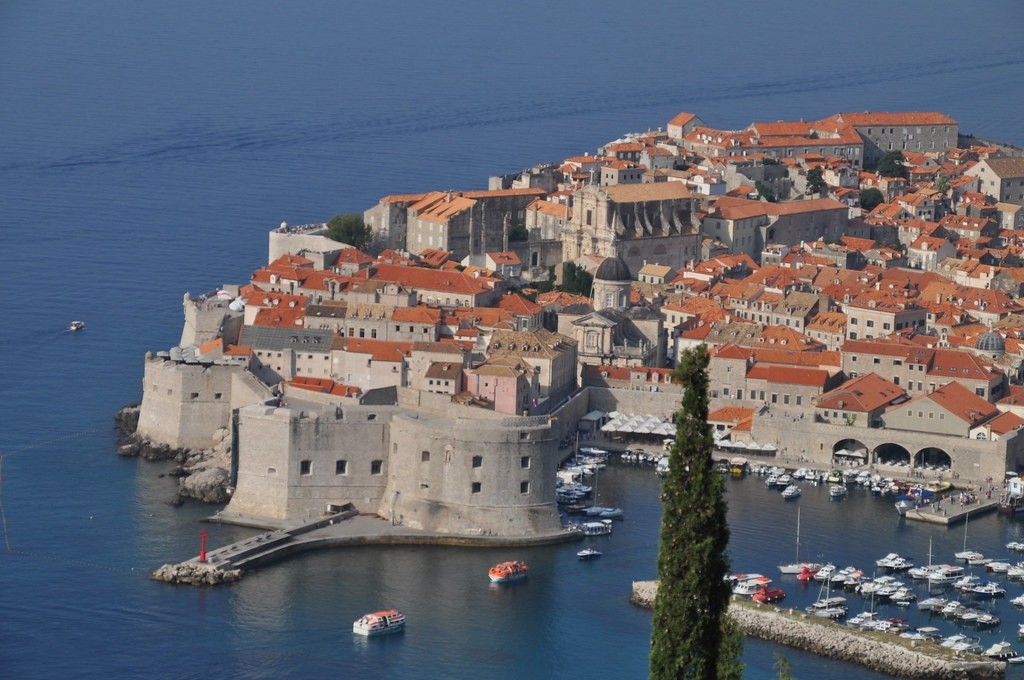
[556,430,624,518]
[352,610,407,637]
[792,467,952,515]
[781,485,802,499]
[717,457,795,485]
[575,519,613,536]
[829,485,847,496]
[621,451,672,478]
[577,548,602,560]
[723,505,1024,665]
[488,560,530,583]
[69,320,85,331]
